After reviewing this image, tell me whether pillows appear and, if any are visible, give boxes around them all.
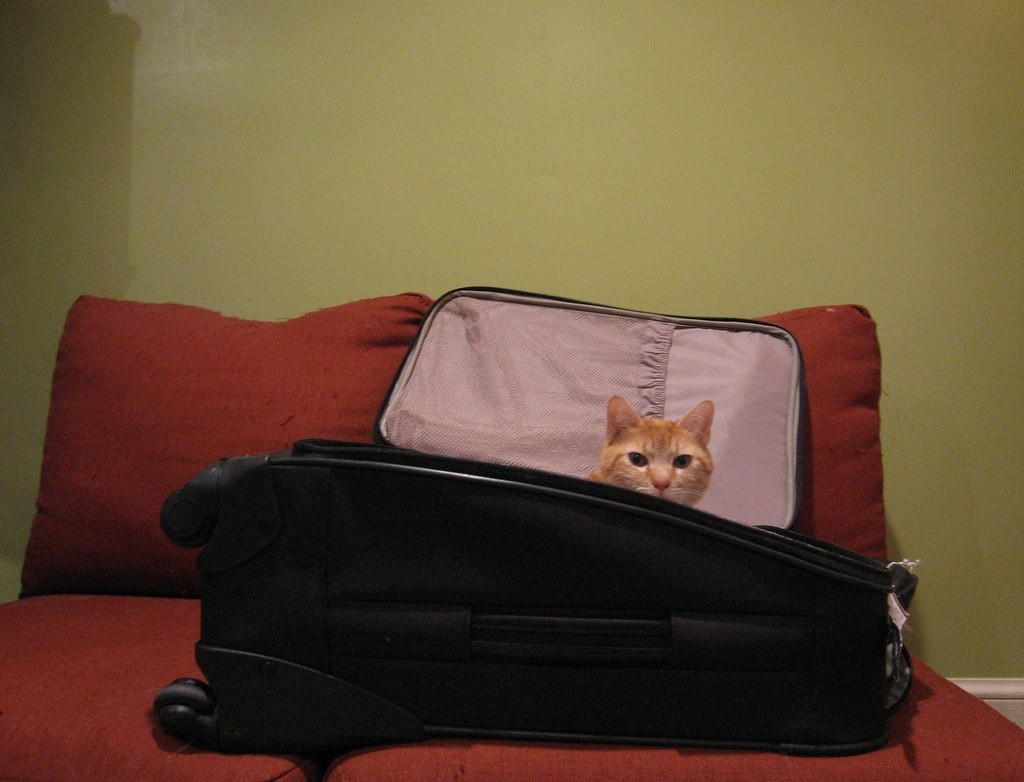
[748,300,895,563]
[20,291,437,594]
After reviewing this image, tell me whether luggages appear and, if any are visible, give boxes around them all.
[154,286,921,757]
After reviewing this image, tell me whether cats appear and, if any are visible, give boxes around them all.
[582,395,714,508]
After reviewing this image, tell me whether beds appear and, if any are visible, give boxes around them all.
[1,568,1024,781]
